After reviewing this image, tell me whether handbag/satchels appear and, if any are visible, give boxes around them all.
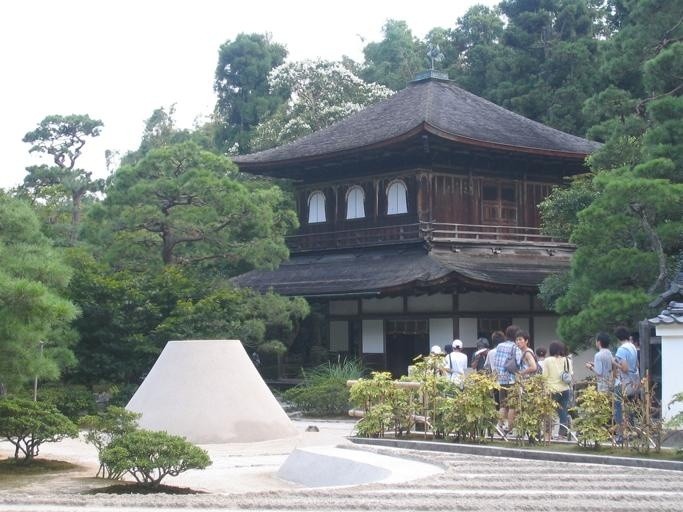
[503,353,520,375]
[619,368,641,399]
[450,372,464,390]
[476,358,485,372]
[561,371,572,383]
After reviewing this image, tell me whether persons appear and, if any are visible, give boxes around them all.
[427,324,648,445]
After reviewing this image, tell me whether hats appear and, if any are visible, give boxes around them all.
[429,345,444,355]
[451,339,462,348]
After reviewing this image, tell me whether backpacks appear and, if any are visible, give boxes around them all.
[520,349,542,377]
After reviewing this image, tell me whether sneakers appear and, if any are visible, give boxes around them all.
[608,435,632,444]
[503,426,513,435]
